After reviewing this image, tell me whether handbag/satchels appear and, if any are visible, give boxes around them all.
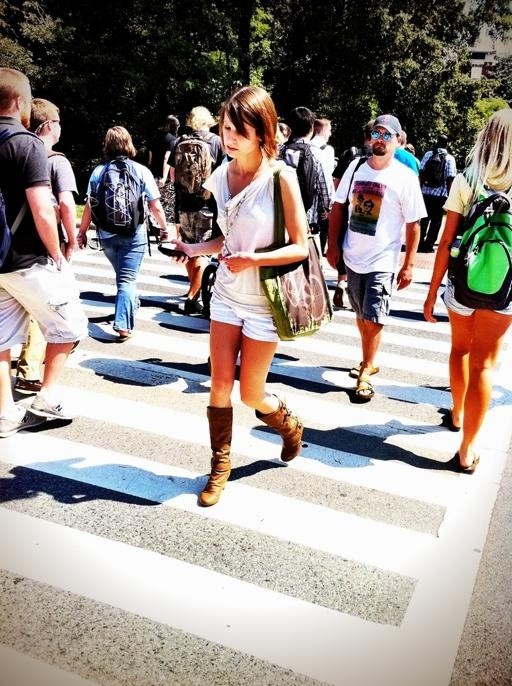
[254,162,334,343]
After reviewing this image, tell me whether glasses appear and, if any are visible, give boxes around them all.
[369,130,399,143]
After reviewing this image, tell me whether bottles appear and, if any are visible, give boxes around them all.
[450,232,462,262]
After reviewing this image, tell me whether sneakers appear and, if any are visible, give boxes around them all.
[333,283,346,308]
[0,404,48,439]
[179,293,206,317]
[27,390,77,421]
[13,376,43,396]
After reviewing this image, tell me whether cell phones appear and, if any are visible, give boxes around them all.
[157,243,190,262]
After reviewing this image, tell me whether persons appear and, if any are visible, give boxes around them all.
[0,66,75,439]
[423,107,512,474]
[274,106,374,312]
[393,128,458,253]
[77,125,168,342]
[324,114,428,397]
[11,96,80,396]
[170,85,309,506]
[147,105,226,315]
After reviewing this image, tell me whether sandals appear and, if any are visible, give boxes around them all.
[116,330,135,343]
[349,360,382,379]
[355,378,375,399]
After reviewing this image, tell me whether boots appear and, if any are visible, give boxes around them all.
[255,393,305,462]
[197,406,234,508]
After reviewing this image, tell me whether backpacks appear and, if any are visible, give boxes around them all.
[0,125,45,274]
[422,149,451,190]
[279,140,318,213]
[89,155,149,237]
[446,165,512,312]
[174,131,217,212]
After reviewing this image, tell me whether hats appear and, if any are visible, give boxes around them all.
[370,113,403,138]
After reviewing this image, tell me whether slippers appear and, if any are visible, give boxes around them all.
[458,447,481,475]
[449,406,462,432]
